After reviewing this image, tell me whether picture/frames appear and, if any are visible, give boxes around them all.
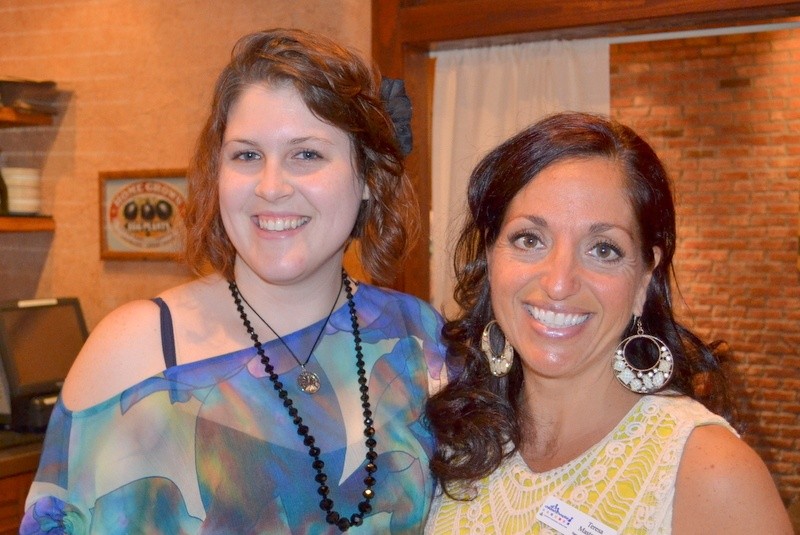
[99,168,189,263]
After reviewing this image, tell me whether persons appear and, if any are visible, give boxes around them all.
[23,25,457,535]
[408,113,797,535]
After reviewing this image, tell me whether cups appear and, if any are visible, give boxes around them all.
[1,167,40,215]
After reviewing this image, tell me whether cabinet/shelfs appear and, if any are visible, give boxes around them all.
[0,106,54,232]
[0,440,44,535]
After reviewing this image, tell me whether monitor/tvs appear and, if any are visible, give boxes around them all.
[0,296,89,397]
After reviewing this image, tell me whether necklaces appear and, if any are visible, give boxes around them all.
[229,267,380,530]
[233,273,348,393]
